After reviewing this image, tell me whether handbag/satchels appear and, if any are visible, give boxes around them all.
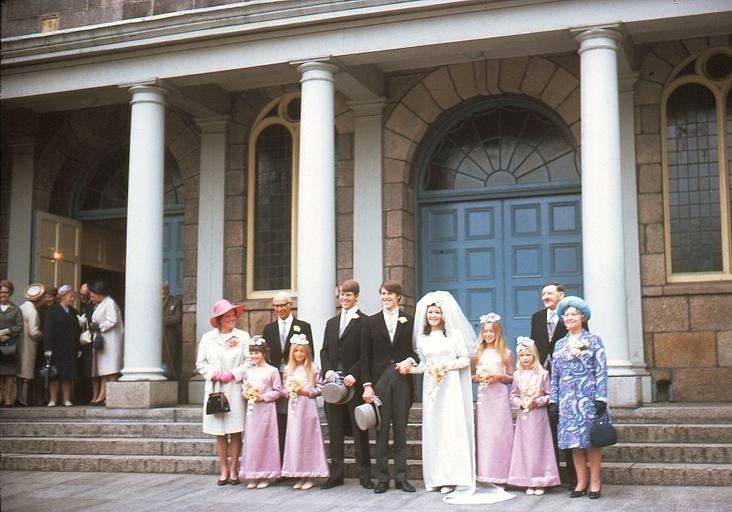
[591,425,618,447]
[37,363,61,382]
[206,392,230,414]
[80,332,102,350]
[0,343,17,358]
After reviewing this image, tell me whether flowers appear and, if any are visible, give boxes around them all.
[478,369,491,391]
[241,383,259,411]
[569,340,590,359]
[287,379,300,402]
[520,383,539,414]
[427,360,447,395]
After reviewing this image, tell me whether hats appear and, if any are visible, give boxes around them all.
[322,377,356,406]
[353,400,382,432]
[0,280,110,298]
[209,300,245,328]
[556,296,589,317]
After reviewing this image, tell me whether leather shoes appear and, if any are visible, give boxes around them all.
[360,478,416,493]
[1,397,106,406]
[440,487,453,493]
[218,473,273,488]
[294,475,344,489]
[527,488,544,496]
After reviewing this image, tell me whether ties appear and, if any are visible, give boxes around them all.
[339,312,347,337]
[385,313,393,338]
[280,322,287,352]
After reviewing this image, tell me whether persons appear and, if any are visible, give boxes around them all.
[239,335,282,490]
[527,282,588,490]
[160,277,182,374]
[469,313,514,485]
[1,277,125,407]
[548,296,612,499]
[282,333,331,490]
[318,279,376,490]
[195,300,250,485]
[262,294,314,466]
[395,290,479,494]
[507,335,561,496]
[358,278,421,492]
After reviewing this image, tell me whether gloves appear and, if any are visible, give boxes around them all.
[77,313,88,327]
[550,404,558,422]
[594,401,607,416]
[211,371,220,381]
[0,329,11,341]
[221,372,234,384]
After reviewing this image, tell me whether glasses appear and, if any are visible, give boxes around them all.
[273,302,289,308]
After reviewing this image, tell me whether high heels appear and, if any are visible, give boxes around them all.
[588,481,601,499]
[569,481,588,496]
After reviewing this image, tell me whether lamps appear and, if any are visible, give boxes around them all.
[464,49,484,60]
